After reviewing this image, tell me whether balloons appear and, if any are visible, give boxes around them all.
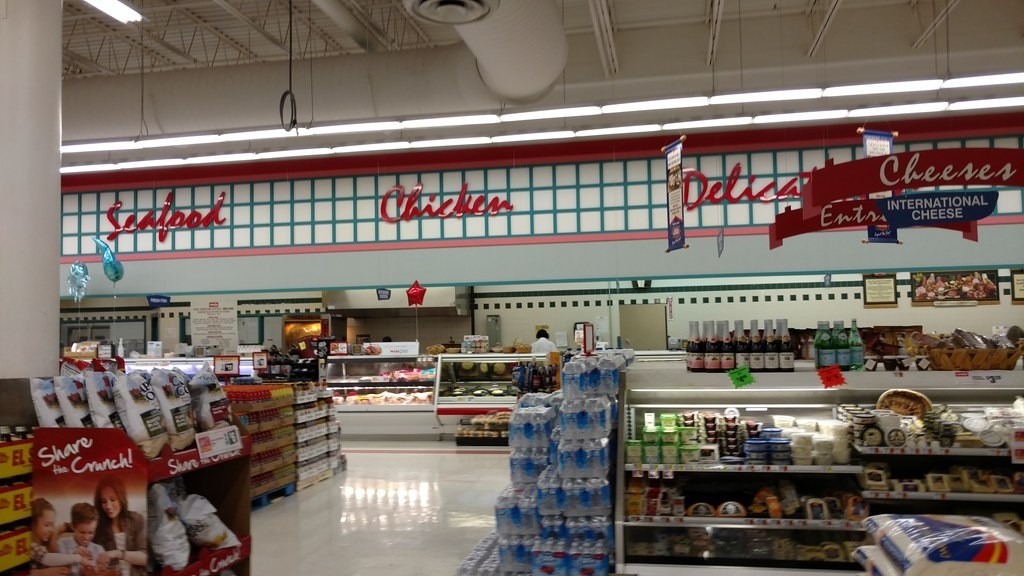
[93,237,124,282]
[66,261,90,303]
[406,280,427,306]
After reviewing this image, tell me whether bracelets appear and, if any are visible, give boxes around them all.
[120,550,125,560]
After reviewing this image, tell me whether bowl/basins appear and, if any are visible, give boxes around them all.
[445,348,461,353]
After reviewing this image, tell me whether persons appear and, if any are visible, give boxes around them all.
[532,329,556,360]
[1006,326,1024,347]
[48,474,148,576]
[57,503,105,576]
[374,336,399,372]
[30,498,97,569]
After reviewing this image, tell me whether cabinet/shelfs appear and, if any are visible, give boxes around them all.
[324,355,441,439]
[0,438,33,574]
[614,362,1024,576]
[31,426,252,576]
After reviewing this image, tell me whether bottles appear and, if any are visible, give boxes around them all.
[455,348,637,576]
[685,318,795,374]
[812,320,849,370]
[220,380,340,488]
[848,318,865,370]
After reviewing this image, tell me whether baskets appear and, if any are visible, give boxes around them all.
[517,343,533,353]
[876,389,934,421]
[504,346,516,353]
[928,348,1024,371]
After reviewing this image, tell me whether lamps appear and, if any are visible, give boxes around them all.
[83,0,151,25]
